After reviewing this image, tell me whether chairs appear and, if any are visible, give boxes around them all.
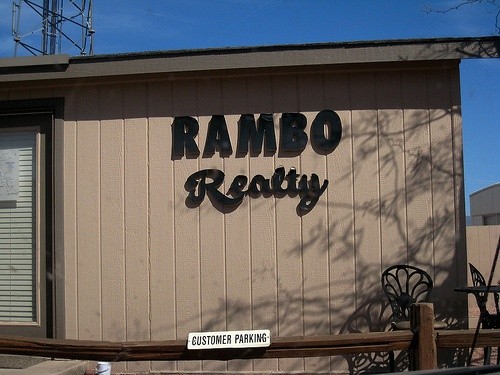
[380,264,448,372]
[468,262,500,366]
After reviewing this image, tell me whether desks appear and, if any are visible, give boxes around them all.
[454,285,500,365]
